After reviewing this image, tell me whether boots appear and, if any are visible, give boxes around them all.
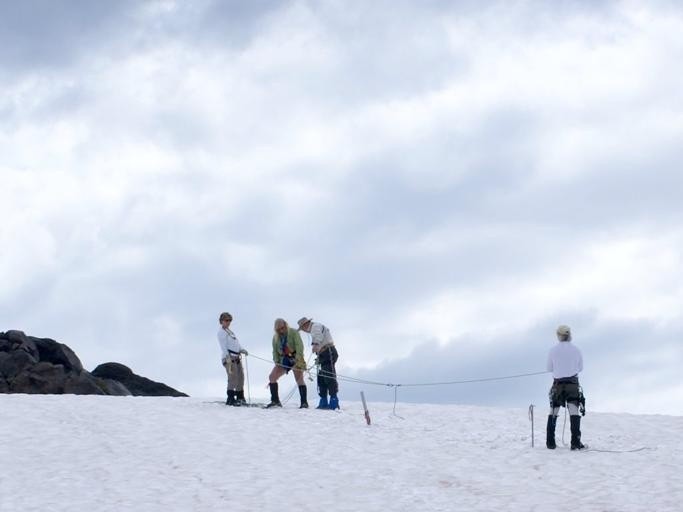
[263,382,282,409]
[237,391,249,406]
[570,415,584,450]
[315,398,328,409]
[329,396,340,410]
[298,385,309,409]
[546,414,558,449]
[226,389,240,407]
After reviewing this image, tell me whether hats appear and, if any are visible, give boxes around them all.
[297,316,312,332]
[557,325,570,336]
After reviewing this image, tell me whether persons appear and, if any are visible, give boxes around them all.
[544,324,589,451]
[295,317,342,410]
[260,318,309,409]
[216,312,250,407]
